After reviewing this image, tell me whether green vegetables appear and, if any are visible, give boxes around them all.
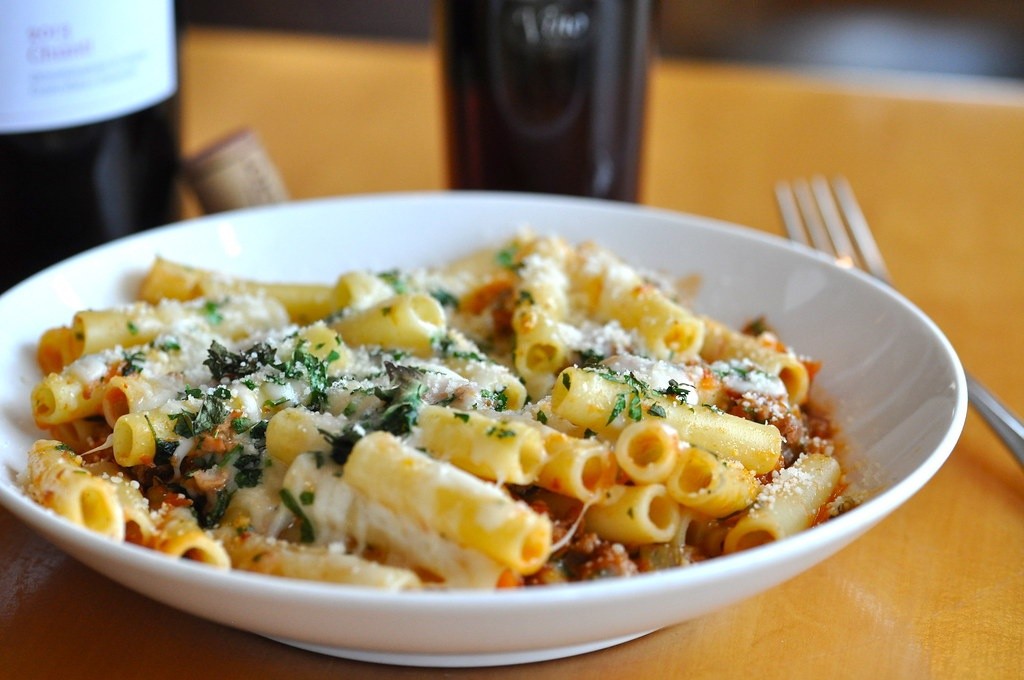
[52,248,699,546]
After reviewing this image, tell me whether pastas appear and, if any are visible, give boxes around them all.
[26,229,844,590]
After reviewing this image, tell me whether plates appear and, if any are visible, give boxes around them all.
[0,186,970,670]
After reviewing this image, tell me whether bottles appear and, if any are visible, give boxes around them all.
[0,0,187,292]
[432,0,659,204]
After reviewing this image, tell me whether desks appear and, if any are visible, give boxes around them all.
[0,34,1024,680]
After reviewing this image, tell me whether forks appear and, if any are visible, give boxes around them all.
[770,175,1024,469]
